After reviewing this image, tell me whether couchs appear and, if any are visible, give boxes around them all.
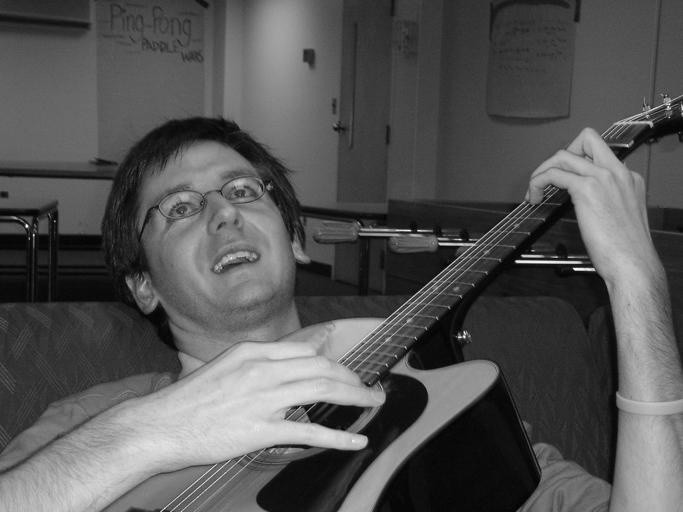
[2,294,617,512]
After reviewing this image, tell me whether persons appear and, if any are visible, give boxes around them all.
[1,111,682,512]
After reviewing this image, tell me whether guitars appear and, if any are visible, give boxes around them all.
[103,94,681,512]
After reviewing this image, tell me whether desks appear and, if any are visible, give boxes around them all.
[1,195,61,308]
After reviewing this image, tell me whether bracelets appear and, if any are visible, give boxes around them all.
[611,389,683,417]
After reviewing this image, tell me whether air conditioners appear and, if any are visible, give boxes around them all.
[1,2,91,34]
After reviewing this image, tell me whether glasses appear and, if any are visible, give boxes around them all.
[134,178,272,242]
